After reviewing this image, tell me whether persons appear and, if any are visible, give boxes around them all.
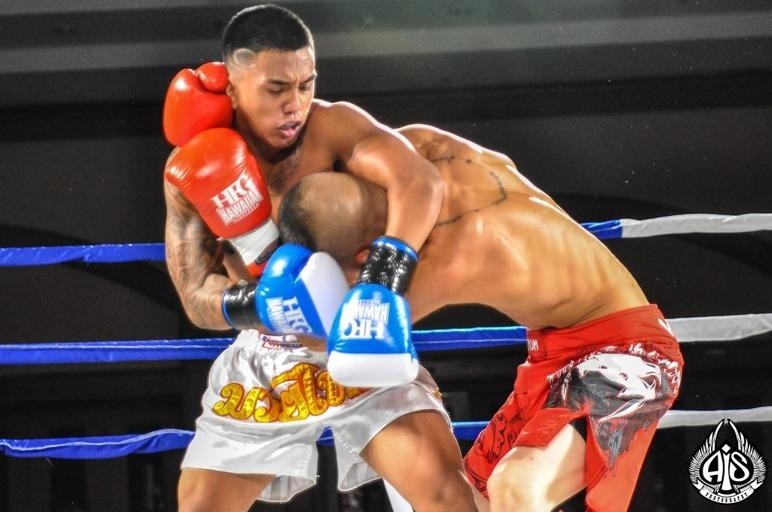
[159,60,686,512]
[162,2,478,510]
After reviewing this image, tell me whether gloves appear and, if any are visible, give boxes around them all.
[324,235,420,390]
[159,60,234,148]
[162,125,282,277]
[220,240,352,341]
